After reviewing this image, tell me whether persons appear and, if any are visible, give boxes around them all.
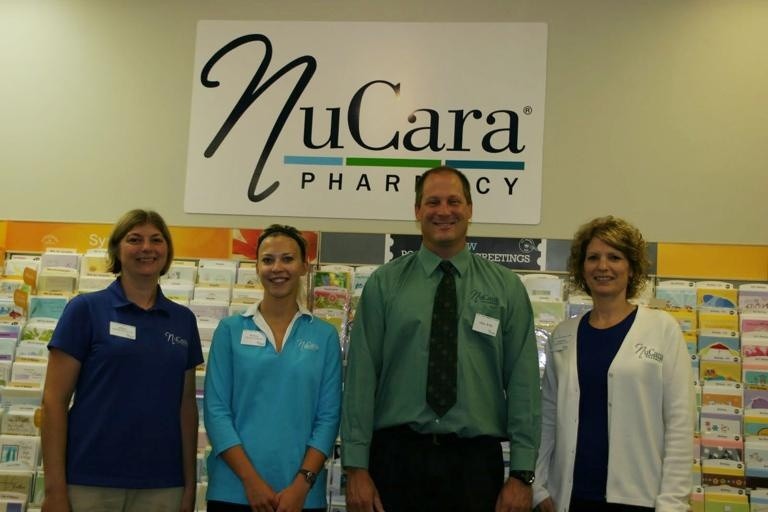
[202,223,345,512]
[339,165,544,512]
[38,207,204,511]
[530,215,697,512]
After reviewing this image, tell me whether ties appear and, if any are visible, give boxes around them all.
[426,259,457,417]
